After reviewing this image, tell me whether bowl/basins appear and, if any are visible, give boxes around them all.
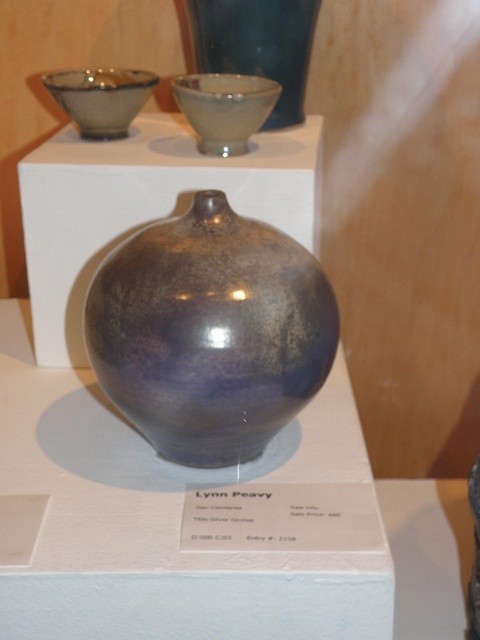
[168,74,283,157]
[39,68,160,140]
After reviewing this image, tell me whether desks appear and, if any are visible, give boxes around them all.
[0,296,398,636]
